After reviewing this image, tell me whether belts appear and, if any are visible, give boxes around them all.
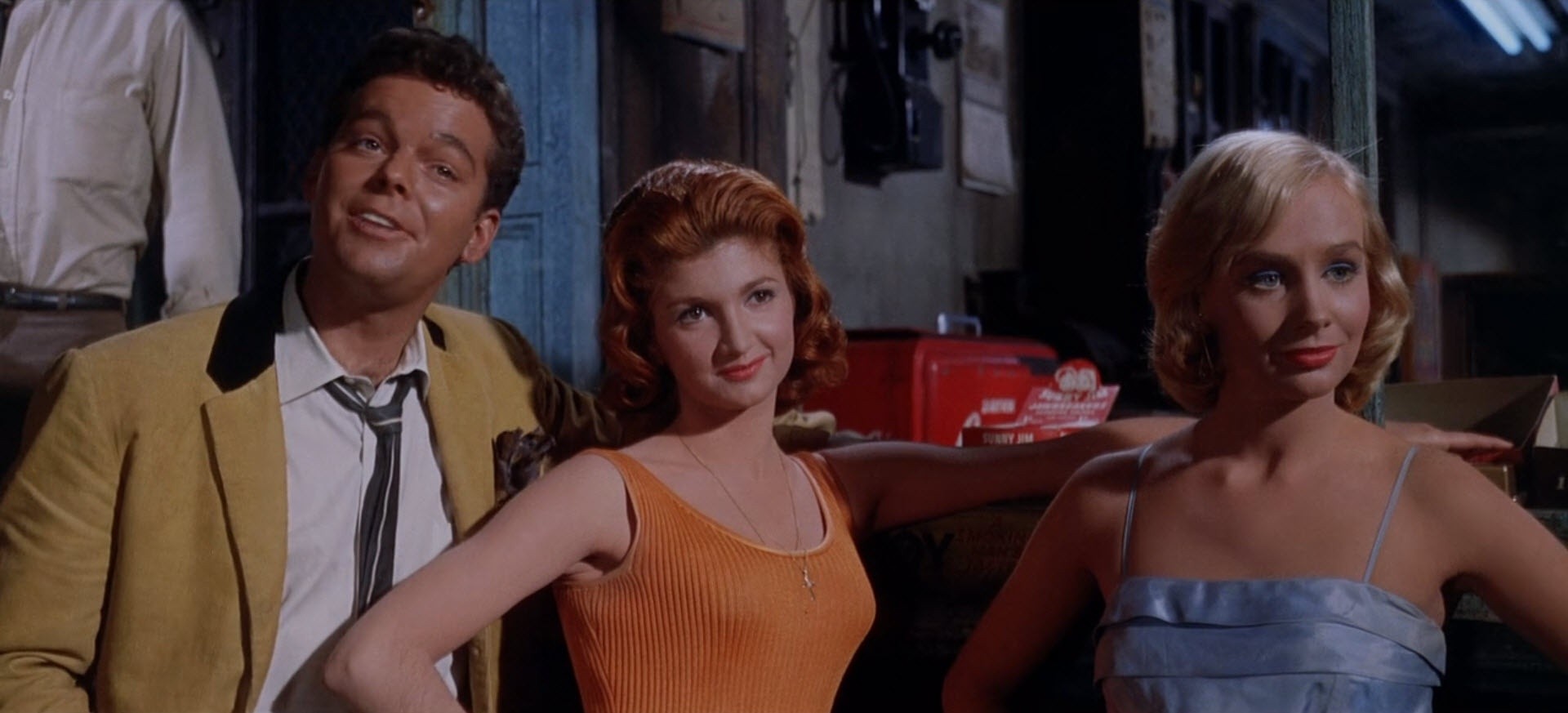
[0,284,125,309]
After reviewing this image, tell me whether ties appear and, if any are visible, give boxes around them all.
[319,366,416,616]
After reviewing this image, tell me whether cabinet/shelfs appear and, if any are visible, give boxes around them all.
[1004,0,1398,413]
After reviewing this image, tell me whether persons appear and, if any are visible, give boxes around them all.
[0,0,243,495]
[327,158,1511,713]
[941,131,1568,713]
[0,23,876,713]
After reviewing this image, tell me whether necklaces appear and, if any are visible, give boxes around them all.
[676,426,816,601]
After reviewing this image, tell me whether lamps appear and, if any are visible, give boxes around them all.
[1458,0,1559,56]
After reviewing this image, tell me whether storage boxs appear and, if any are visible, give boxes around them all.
[1380,372,1568,509]
